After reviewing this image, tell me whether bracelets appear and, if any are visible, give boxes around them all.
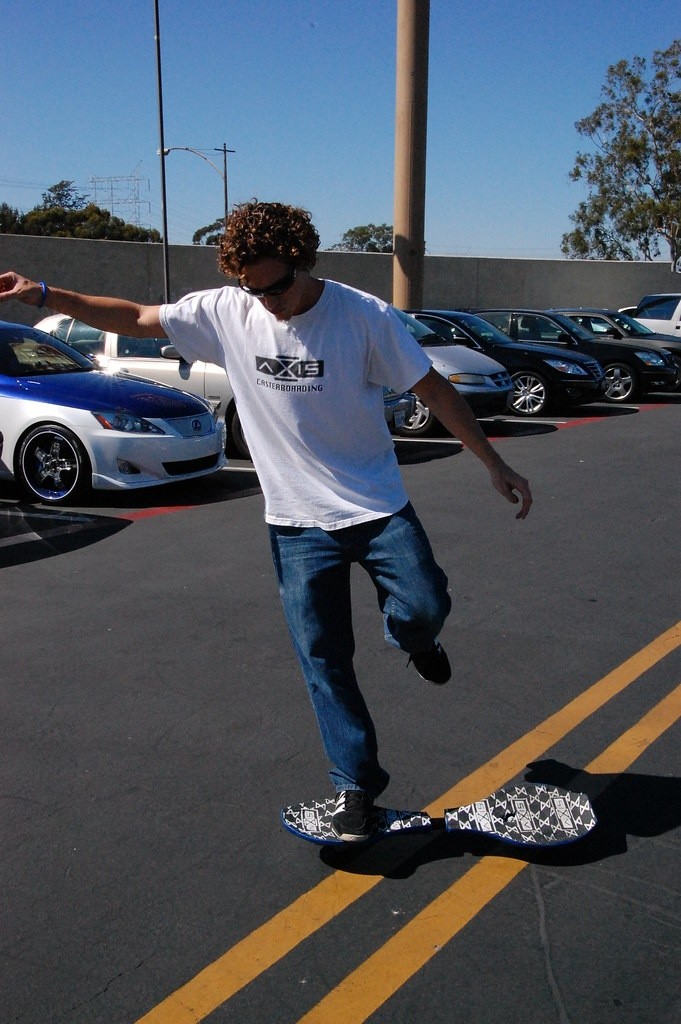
[37,282,47,309]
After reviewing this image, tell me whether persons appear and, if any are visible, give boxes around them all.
[0,201,533,842]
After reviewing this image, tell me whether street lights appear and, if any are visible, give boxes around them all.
[156,146,229,232]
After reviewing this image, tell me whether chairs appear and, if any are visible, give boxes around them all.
[528,320,541,340]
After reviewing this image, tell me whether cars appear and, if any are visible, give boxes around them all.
[462,308,681,405]
[501,310,681,394]
[0,321,229,508]
[32,312,416,464]
[389,308,517,439]
[423,310,608,419]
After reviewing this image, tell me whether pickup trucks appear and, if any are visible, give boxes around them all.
[579,293,680,338]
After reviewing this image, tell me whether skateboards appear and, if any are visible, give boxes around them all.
[277,785,599,853]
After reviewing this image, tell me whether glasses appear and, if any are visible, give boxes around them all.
[238,267,296,299]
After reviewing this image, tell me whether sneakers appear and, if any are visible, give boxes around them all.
[330,791,375,842]
[404,638,452,685]
